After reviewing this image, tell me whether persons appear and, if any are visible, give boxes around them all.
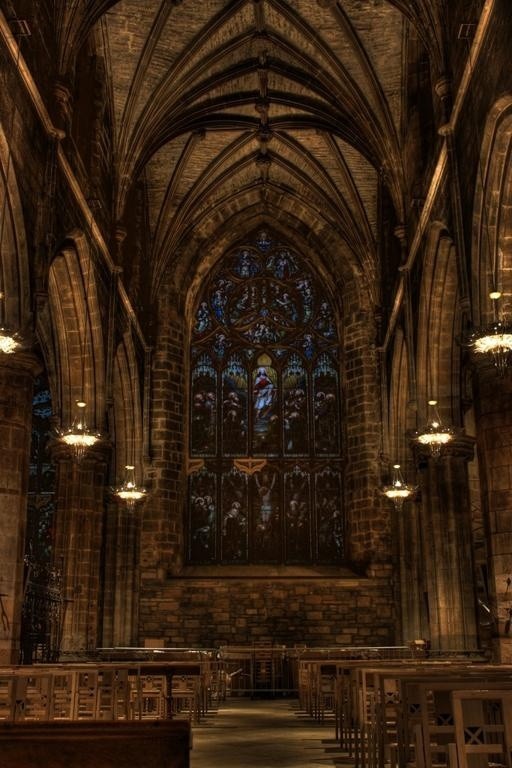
[187,465,342,561]
[189,371,339,454]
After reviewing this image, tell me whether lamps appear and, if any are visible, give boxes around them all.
[415,220,458,457]
[468,158,512,375]
[113,361,147,512]
[0,41,31,355]
[385,465,415,511]
[60,218,102,462]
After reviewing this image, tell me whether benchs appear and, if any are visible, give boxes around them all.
[300,656,512,768]
[0,656,228,726]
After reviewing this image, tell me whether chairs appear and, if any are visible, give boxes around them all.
[252,640,274,684]
